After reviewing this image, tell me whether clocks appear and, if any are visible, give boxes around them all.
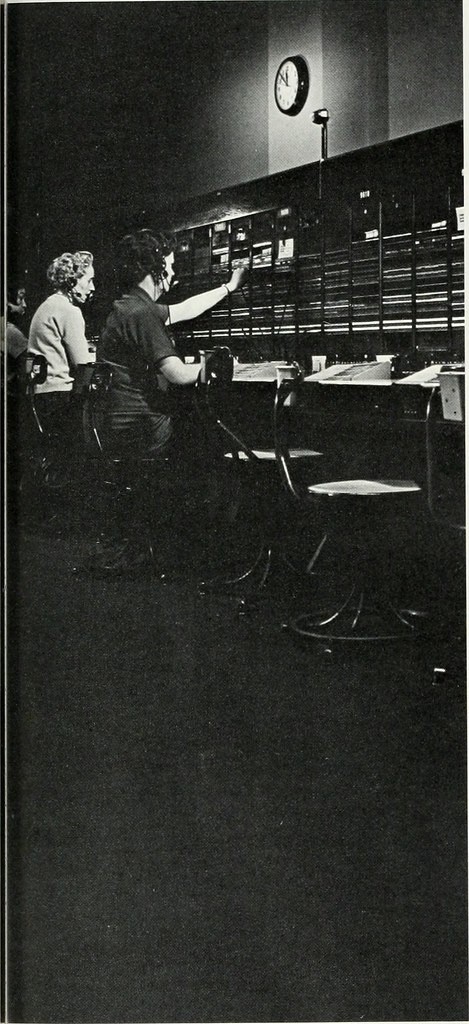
[274,56,310,117]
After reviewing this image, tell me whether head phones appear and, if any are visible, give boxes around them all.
[149,230,169,285]
[66,277,79,287]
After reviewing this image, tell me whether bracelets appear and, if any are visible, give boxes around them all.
[222,284,230,295]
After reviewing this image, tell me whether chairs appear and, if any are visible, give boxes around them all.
[9,354,90,542]
[193,345,339,611]
[70,362,193,586]
[274,376,448,643]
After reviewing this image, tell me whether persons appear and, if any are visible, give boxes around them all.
[0,280,27,400]
[95,228,249,465]
[27,251,95,433]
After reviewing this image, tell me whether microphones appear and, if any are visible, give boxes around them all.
[74,288,82,298]
[165,276,174,290]
